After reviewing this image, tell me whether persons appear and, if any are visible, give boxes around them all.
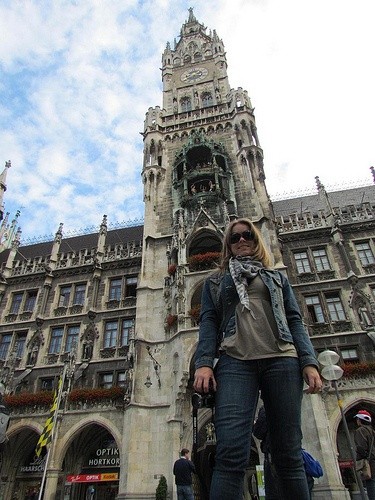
[353,408,375,500]
[253,403,324,500]
[173,448,197,500]
[193,218,324,500]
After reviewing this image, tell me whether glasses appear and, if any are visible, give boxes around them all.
[231,231,254,244]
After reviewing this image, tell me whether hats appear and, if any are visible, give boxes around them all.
[353,410,372,423]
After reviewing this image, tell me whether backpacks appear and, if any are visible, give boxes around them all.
[301,448,323,478]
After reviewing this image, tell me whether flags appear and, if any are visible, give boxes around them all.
[34,374,63,458]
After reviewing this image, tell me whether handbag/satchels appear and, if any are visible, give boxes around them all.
[356,458,372,481]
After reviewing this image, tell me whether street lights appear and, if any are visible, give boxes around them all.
[318,349,367,500]
